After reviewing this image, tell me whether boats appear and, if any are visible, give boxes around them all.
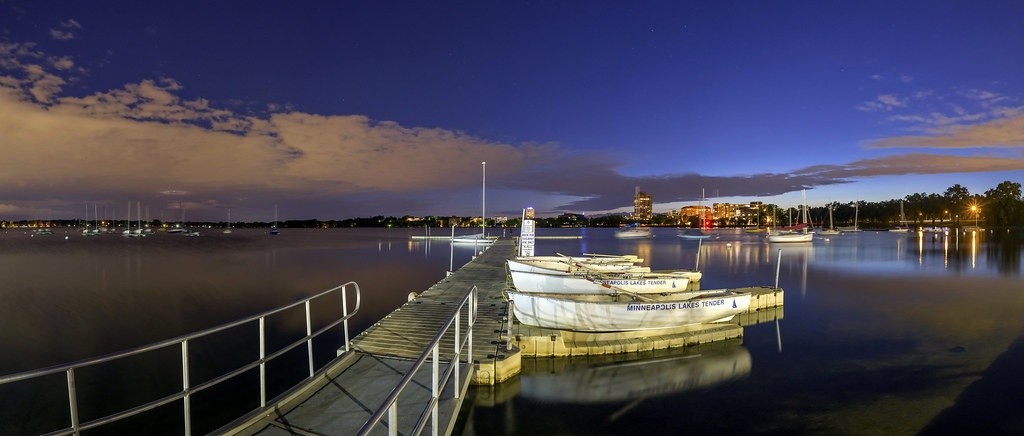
[507,259,690,294]
[506,290,752,332]
[516,255,635,270]
[888,226,910,232]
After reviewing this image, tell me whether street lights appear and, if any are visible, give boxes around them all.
[482,162,486,239]
[972,206,977,226]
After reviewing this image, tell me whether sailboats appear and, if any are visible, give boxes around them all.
[269,202,280,235]
[744,184,815,242]
[816,203,840,235]
[32,201,200,237]
[677,185,711,239]
[614,186,651,238]
[222,209,233,233]
[842,200,862,232]
